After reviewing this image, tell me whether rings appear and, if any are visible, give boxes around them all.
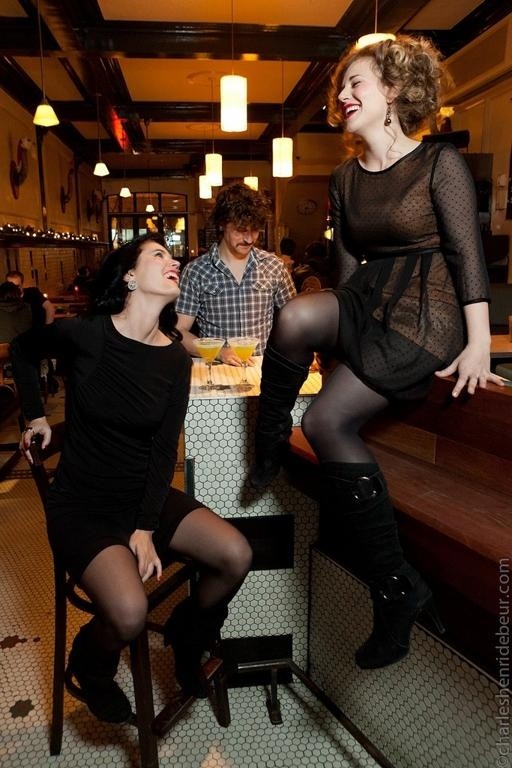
[24,426,35,434]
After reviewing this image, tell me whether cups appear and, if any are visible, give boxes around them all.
[227,337,260,391]
[192,337,226,390]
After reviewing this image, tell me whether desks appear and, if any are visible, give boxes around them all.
[185,353,326,679]
[41,288,91,308]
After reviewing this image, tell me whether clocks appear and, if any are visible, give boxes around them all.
[299,199,315,215]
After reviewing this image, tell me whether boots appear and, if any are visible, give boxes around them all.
[252,343,309,489]
[319,460,449,670]
[68,627,132,724]
[163,595,228,698]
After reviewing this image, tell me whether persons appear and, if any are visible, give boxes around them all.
[70,265,91,290]
[7,270,53,364]
[14,238,252,723]
[278,237,294,271]
[0,280,31,345]
[170,184,298,366]
[288,241,331,293]
[247,33,505,669]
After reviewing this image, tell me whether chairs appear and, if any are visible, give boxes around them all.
[0,342,28,435]
[29,420,233,768]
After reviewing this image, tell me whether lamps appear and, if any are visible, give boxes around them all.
[271,137,294,179]
[356,33,398,47]
[220,75,249,132]
[244,177,259,193]
[94,162,110,177]
[119,187,131,199]
[144,204,155,212]
[199,176,212,198]
[205,153,223,187]
[33,99,60,128]
